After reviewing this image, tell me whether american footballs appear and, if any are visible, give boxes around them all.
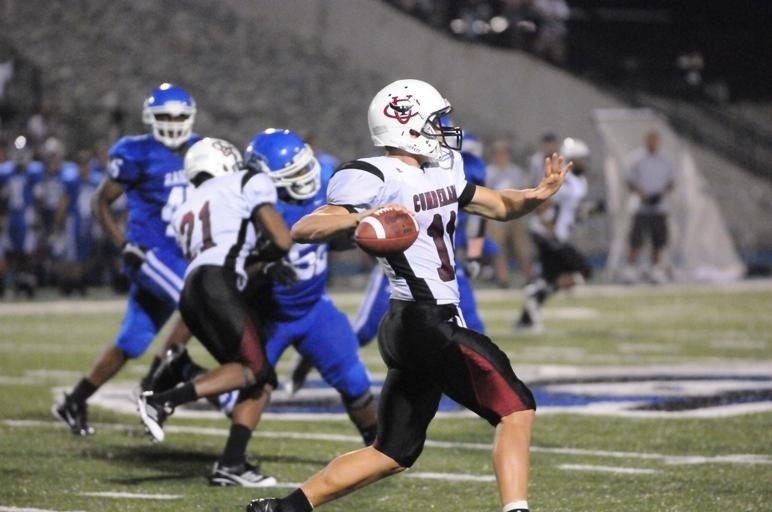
[354,207,419,256]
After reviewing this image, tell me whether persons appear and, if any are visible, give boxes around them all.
[0,135,130,296]
[447,1,571,66]
[622,130,676,288]
[482,133,605,331]
[245,78,572,511]
[51,82,378,487]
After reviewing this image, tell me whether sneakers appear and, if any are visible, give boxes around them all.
[210,460,276,486]
[246,498,281,512]
[132,343,187,443]
[52,396,94,436]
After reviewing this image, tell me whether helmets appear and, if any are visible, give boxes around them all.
[184,137,243,182]
[245,128,321,200]
[142,85,196,148]
[367,79,462,163]
